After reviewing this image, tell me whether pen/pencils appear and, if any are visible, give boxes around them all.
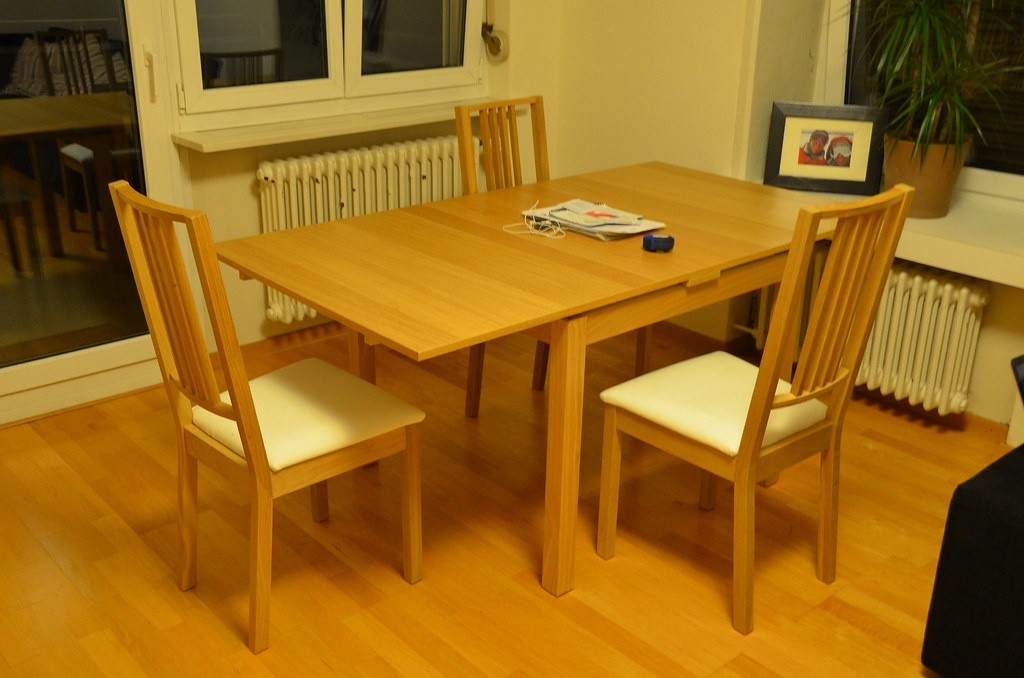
[586,210,619,218]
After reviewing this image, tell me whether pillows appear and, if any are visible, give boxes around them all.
[5,34,132,99]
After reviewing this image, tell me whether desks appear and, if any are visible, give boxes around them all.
[0,94,137,281]
[212,162,837,598]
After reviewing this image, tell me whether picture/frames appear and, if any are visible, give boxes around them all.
[763,102,888,196]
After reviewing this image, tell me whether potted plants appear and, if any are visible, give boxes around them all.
[852,0,1024,220]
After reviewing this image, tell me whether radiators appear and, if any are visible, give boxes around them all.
[257,135,482,325]
[757,250,985,415]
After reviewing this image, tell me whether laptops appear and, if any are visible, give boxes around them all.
[1011,354,1024,405]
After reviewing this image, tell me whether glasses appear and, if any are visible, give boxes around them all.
[830,144,852,158]
[811,135,828,145]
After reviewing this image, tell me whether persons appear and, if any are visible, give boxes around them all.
[797,129,852,166]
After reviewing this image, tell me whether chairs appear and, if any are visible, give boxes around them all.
[454,96,652,420]
[110,179,426,655]
[202,48,285,88]
[35,29,149,252]
[596,184,915,637]
